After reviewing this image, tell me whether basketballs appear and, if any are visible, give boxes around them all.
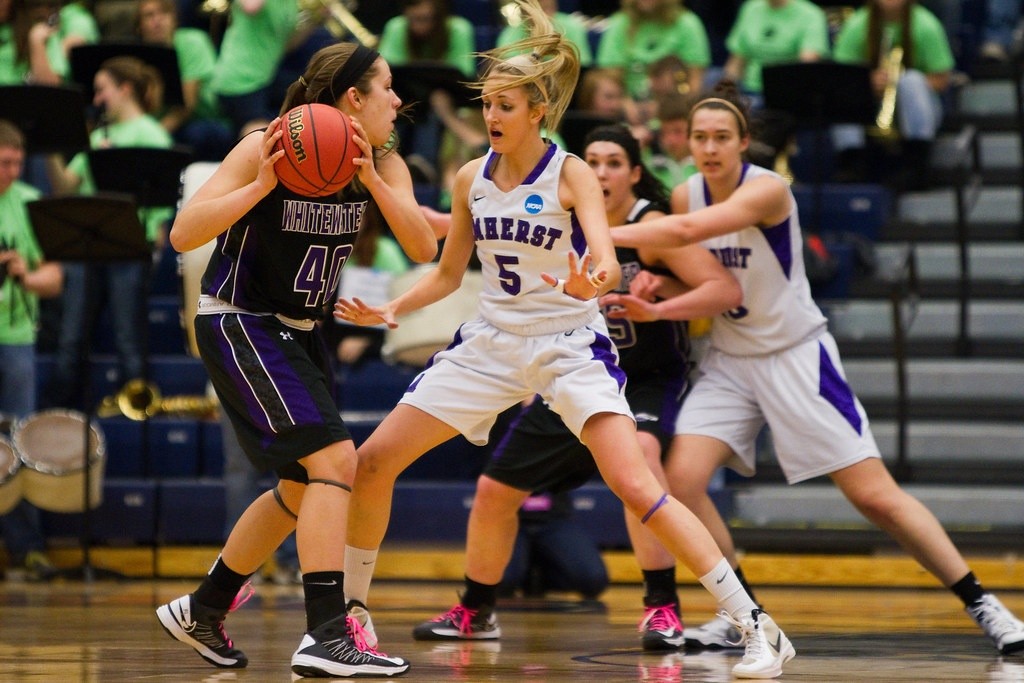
[269,103,362,197]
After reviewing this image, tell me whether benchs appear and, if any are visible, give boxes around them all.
[0,0,1017,546]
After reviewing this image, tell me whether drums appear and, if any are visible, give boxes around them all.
[0,432,23,515]
[12,409,104,511]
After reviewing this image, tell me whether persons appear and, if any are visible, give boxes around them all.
[0,0,954,413]
[343,0,796,681]
[0,119,66,573]
[155,42,438,677]
[607,80,1024,654]
[411,123,744,649]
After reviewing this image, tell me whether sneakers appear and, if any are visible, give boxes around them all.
[637,595,686,650]
[412,605,501,639]
[684,615,743,647]
[156,593,249,667]
[966,594,1024,653]
[346,599,378,648]
[291,614,410,677]
[717,606,796,679]
